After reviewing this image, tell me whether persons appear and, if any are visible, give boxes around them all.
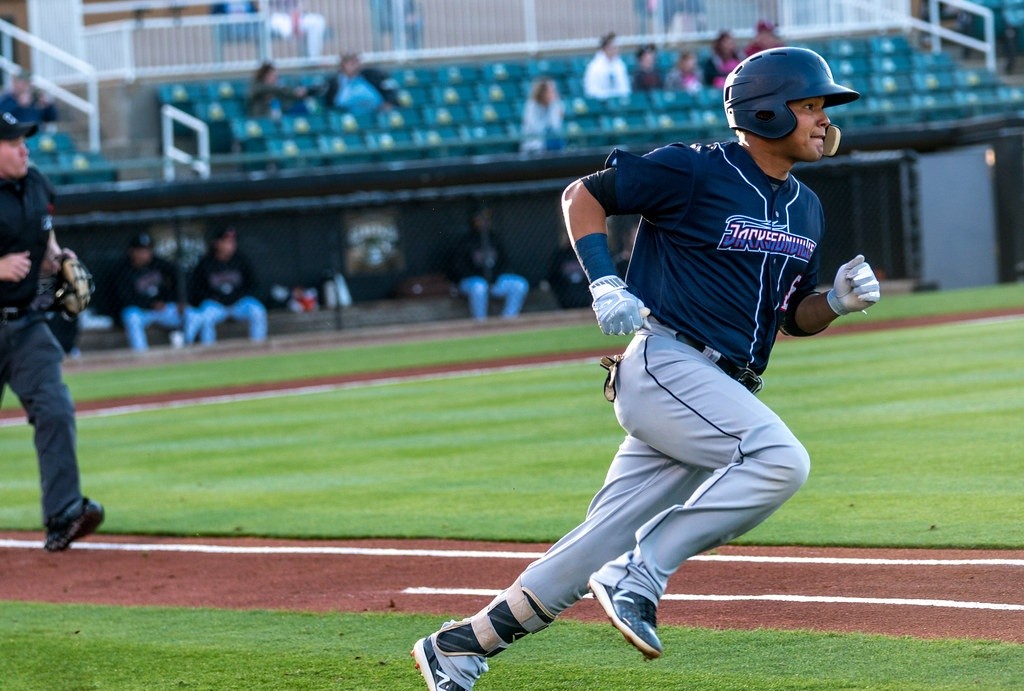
[636,0,705,34]
[291,53,402,116]
[0,71,57,123]
[410,47,880,691]
[548,224,639,311]
[112,230,202,348]
[583,34,630,99]
[209,0,325,62]
[0,110,106,553]
[370,0,422,52]
[669,50,701,94]
[521,77,565,151]
[704,31,744,88]
[246,64,297,116]
[743,20,785,57]
[189,221,269,343]
[633,43,663,88]
[451,206,530,320]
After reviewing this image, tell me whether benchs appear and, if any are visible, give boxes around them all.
[66,277,590,349]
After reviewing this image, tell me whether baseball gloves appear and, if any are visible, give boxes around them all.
[55,249,96,317]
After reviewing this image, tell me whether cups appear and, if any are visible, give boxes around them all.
[171,330,183,349]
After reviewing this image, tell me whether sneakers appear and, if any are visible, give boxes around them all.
[585,575,663,660]
[409,633,470,691]
[44,500,104,550]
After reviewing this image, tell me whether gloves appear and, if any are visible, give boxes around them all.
[827,253,882,316]
[589,276,652,336]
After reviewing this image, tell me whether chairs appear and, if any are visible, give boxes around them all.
[0,30,1024,211]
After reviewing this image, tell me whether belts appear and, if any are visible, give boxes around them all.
[677,331,760,394]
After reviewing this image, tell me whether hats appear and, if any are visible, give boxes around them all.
[134,234,152,250]
[758,21,775,33]
[0,113,37,141]
[218,225,237,239]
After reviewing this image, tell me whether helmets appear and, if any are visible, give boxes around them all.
[721,53,860,140]
